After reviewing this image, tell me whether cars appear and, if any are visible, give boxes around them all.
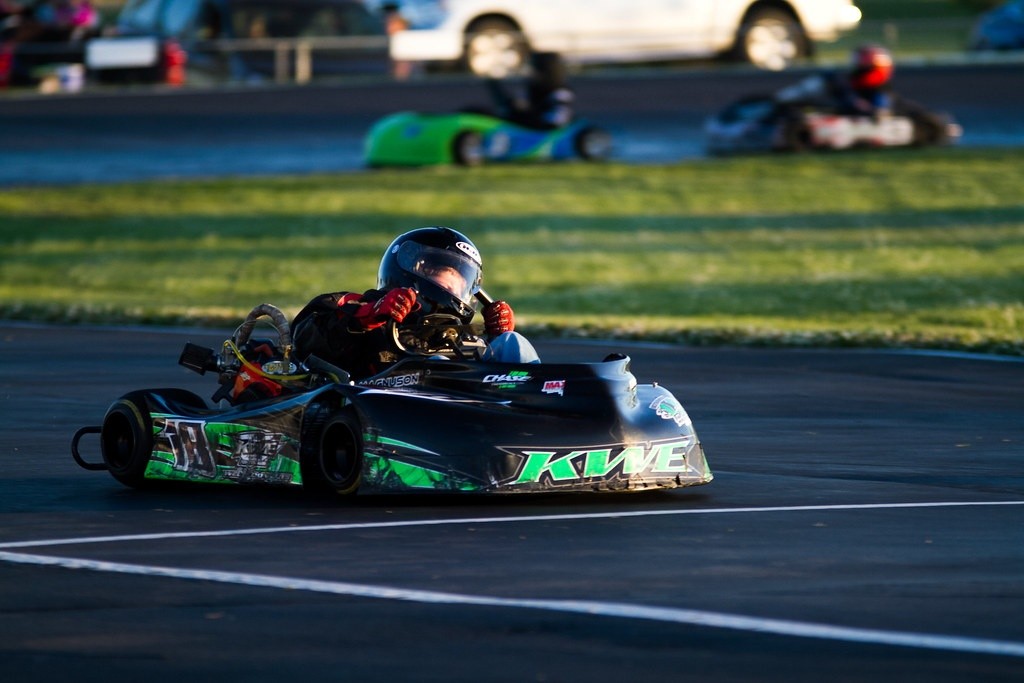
[388,0,868,83]
[967,0,1024,52]
[0,0,389,88]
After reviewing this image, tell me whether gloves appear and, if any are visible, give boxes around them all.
[354,287,417,332]
[481,300,516,336]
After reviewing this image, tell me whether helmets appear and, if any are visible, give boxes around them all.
[552,87,578,105]
[850,46,894,88]
[376,226,484,324]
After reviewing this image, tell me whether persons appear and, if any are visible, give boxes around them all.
[290,226,542,383]
